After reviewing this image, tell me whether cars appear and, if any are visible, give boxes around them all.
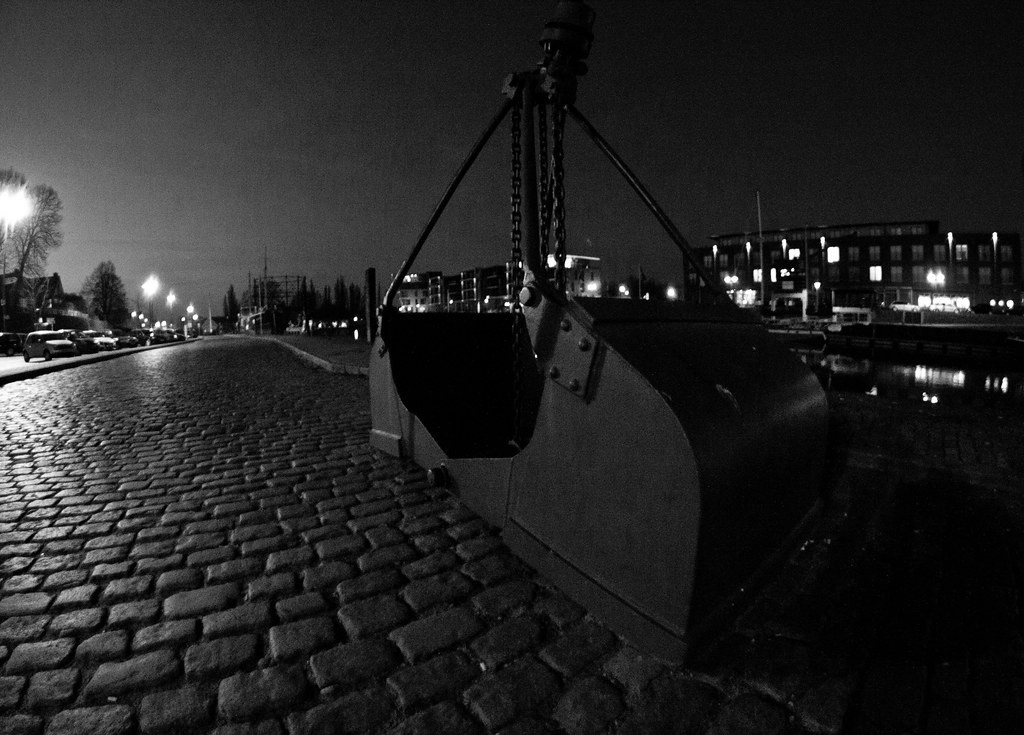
[105,329,138,348]
[152,328,199,343]
[23,330,76,362]
[0,332,22,356]
[82,331,117,351]
[204,326,224,335]
[131,330,158,346]
[991,292,1024,312]
[880,300,920,312]
[971,304,991,314]
[59,329,100,354]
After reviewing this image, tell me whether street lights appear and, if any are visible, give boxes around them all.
[145,282,157,323]
[813,281,821,312]
